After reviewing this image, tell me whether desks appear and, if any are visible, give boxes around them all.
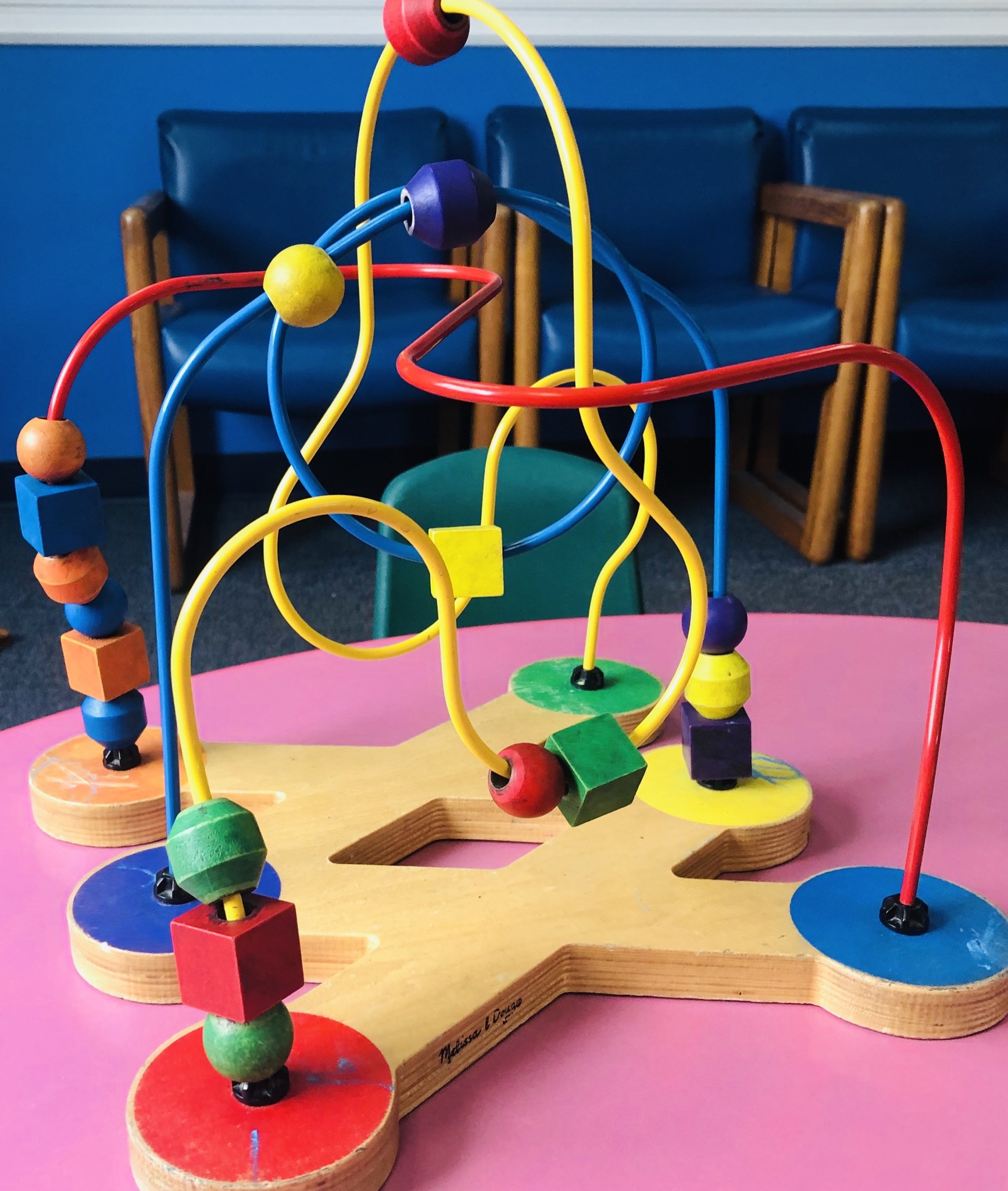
[0,613,1008,1191]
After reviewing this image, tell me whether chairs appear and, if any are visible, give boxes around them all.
[120,106,1008,640]
